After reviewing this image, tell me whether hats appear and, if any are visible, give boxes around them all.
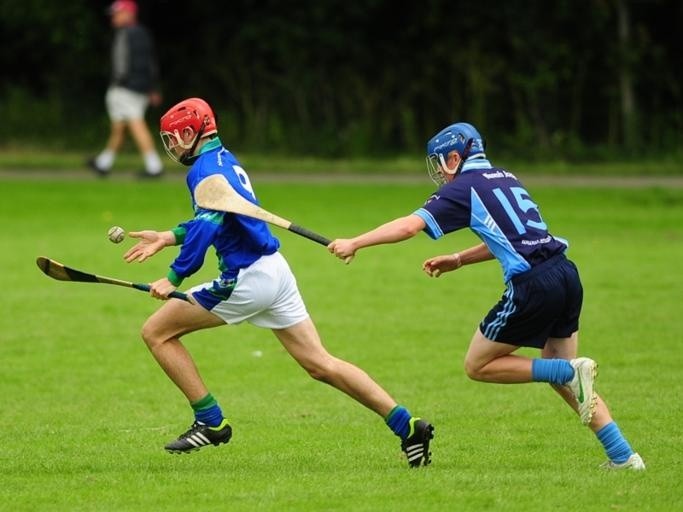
[102,0,138,15]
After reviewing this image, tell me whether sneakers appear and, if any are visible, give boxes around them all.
[83,160,112,178]
[597,452,646,472]
[165,416,233,456]
[134,164,167,180]
[400,416,434,469]
[563,357,597,426]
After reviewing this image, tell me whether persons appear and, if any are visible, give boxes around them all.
[123,97,434,470]
[326,121,648,471]
[87,0,166,182]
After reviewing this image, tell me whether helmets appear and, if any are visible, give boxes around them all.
[158,97,220,149]
[426,121,486,175]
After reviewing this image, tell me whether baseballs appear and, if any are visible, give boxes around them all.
[108,226,125,243]
